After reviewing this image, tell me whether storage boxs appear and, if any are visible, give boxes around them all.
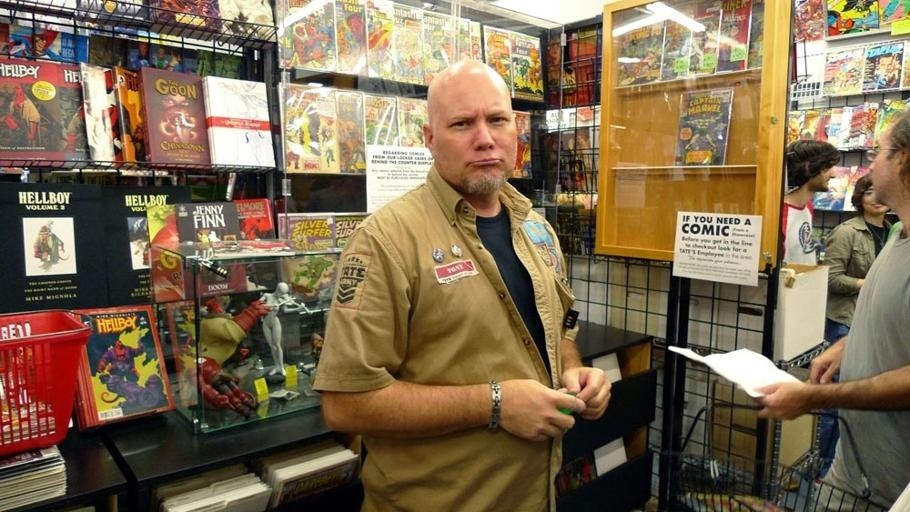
[561,248,837,482]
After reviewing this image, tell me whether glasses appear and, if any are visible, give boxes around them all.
[865,148,900,162]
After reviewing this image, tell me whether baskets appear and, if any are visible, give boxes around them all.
[649,403,890,511]
[0,309,95,458]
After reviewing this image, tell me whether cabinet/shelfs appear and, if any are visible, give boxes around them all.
[596,1,794,277]
[555,318,657,512]
[1,0,284,190]
[1,427,132,512]
[781,79,909,217]
[105,370,368,512]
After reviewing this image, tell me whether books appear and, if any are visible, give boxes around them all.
[675,87,735,165]
[616,0,763,86]
[785,0,910,212]
[153,434,361,512]
[0,444,67,512]
[0,0,602,431]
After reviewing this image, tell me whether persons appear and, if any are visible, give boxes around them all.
[314,57,611,512]
[753,107,910,512]
[814,172,893,490]
[781,139,840,491]
